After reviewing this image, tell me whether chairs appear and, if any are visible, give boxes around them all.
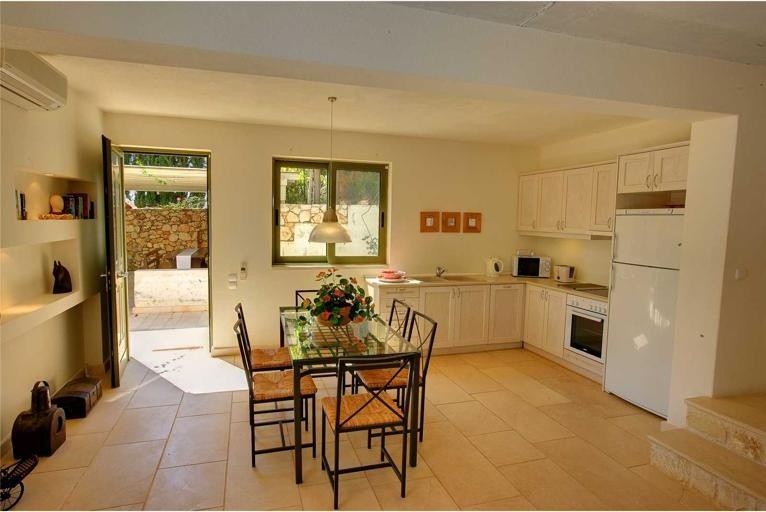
[336,298,412,395]
[233,300,310,432]
[295,289,339,378]
[354,308,439,450]
[233,318,318,469]
[320,348,413,508]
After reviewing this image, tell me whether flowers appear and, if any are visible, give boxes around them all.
[301,266,369,324]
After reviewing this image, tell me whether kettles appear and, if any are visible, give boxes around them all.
[485,255,504,278]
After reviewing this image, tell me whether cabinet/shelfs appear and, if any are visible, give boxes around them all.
[367,276,419,350]
[413,281,490,354]
[523,284,568,362]
[588,159,618,237]
[520,171,540,235]
[541,159,593,239]
[617,144,690,193]
[487,283,526,351]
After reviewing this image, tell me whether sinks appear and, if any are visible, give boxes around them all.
[413,276,446,284]
[442,275,488,283]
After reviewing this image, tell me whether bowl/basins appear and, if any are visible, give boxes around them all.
[377,270,405,279]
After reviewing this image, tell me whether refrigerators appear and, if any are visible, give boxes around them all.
[604,208,684,422]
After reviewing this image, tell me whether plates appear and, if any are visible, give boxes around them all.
[377,278,406,282]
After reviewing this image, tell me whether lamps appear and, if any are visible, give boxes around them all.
[308,95,354,245]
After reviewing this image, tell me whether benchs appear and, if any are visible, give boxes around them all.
[144,249,174,269]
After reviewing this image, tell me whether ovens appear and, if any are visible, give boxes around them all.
[563,304,608,365]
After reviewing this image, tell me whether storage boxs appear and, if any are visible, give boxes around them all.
[51,375,103,419]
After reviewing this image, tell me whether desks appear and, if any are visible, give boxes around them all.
[164,248,208,269]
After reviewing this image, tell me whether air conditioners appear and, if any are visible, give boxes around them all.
[0,46,68,114]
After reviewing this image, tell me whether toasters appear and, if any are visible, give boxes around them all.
[553,265,575,282]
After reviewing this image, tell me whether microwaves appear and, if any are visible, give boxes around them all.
[512,255,551,277]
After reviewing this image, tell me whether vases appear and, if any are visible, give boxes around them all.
[317,302,353,327]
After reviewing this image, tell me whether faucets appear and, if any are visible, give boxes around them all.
[436,265,445,277]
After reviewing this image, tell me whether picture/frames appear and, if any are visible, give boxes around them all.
[463,211,482,232]
[419,209,439,231]
[442,210,460,235]
